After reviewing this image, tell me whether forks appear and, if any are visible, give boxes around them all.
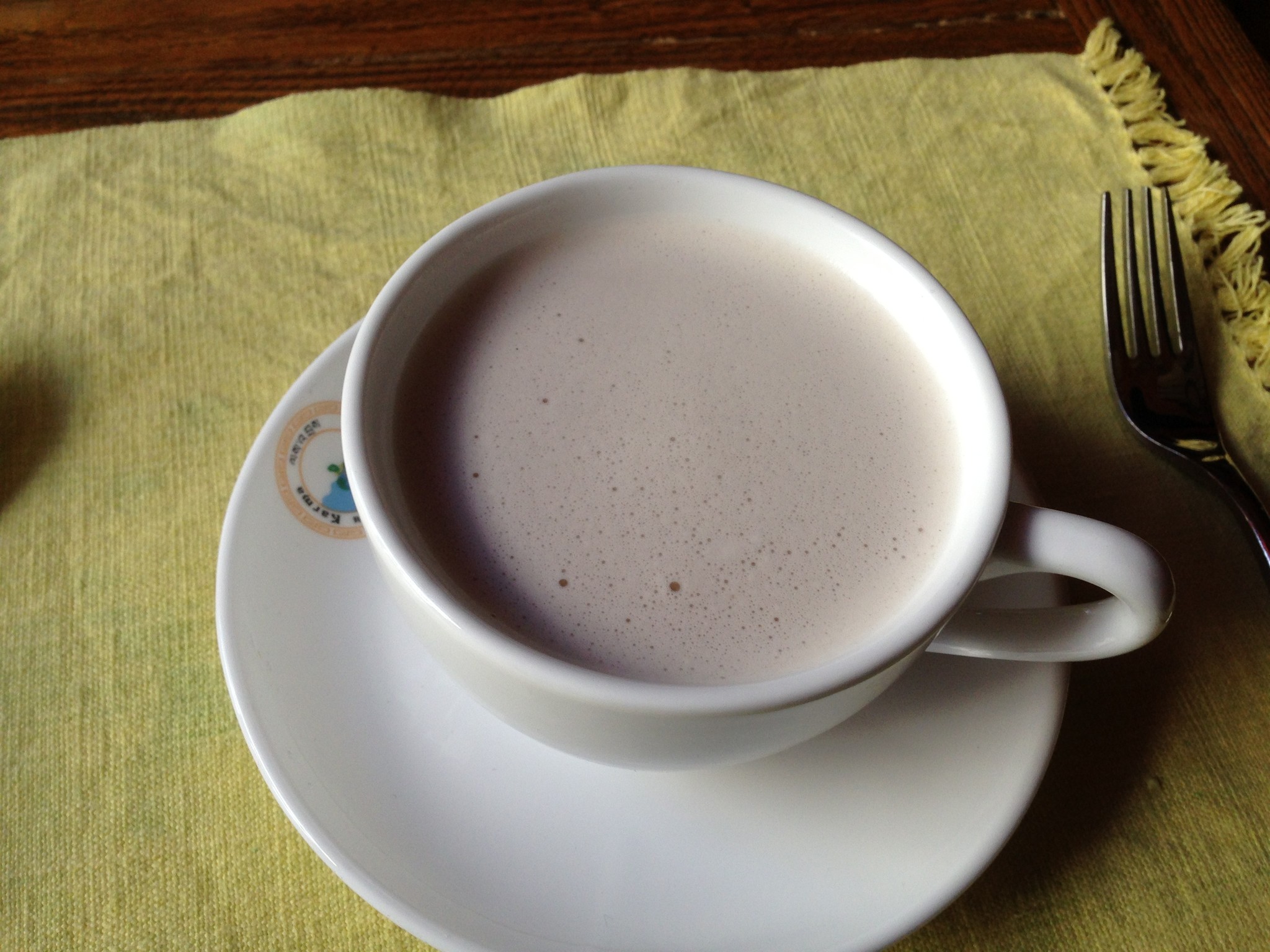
[1097,188,1270,569]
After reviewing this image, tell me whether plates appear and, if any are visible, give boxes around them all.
[215,319,1063,952]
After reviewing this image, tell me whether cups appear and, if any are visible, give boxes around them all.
[338,166,1175,772]
